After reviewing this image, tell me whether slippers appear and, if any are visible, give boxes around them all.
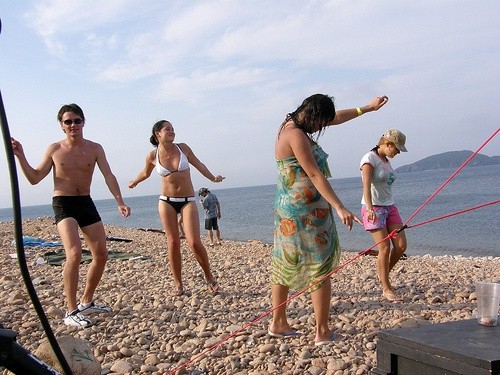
[315,332,346,345]
[268,329,305,338]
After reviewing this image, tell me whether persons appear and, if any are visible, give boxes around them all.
[127,120,226,296]
[198,188,222,249]
[10,104,132,328]
[267,94,390,346]
[359,130,408,302]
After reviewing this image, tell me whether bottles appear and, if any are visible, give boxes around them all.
[36,257,44,264]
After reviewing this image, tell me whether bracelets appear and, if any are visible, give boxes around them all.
[356,107,363,117]
[212,177,217,182]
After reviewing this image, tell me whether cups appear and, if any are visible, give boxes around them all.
[475,282,500,327]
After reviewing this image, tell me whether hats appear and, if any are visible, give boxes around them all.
[199,188,207,196]
[383,129,408,152]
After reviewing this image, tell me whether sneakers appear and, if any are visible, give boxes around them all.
[64,309,92,327]
[78,301,112,315]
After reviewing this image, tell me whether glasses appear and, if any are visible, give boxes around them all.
[62,119,83,125]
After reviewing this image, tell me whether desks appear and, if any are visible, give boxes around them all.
[371,316,500,375]
